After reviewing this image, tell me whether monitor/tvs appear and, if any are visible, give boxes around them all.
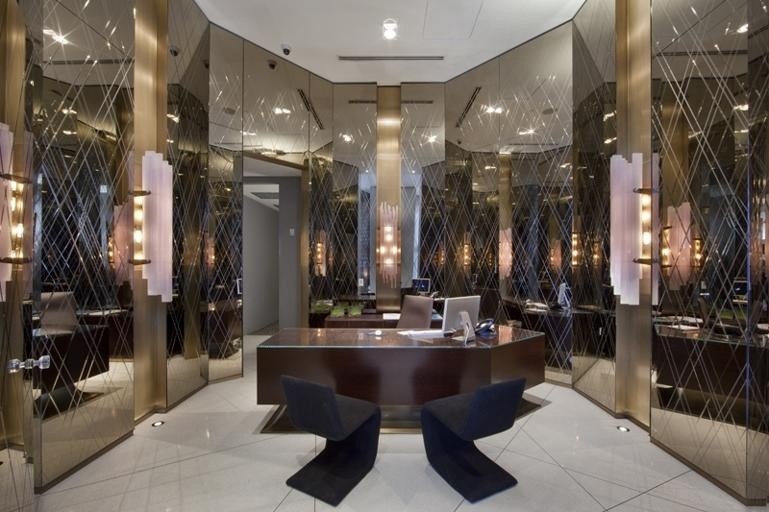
[733,281,751,301]
[442,295,481,343]
[412,278,431,296]
[557,283,570,309]
[236,278,243,295]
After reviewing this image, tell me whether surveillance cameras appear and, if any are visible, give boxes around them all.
[267,58,276,69]
[281,44,291,56]
[457,139,461,144]
[169,47,179,57]
[203,57,209,68]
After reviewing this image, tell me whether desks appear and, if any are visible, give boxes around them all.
[502,296,615,371]
[33,324,110,423]
[255,324,548,436]
[322,309,440,328]
[657,321,769,437]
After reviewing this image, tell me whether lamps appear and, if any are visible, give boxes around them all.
[462,233,472,272]
[107,203,132,288]
[0,120,34,305]
[608,152,660,307]
[663,202,692,292]
[376,201,402,289]
[128,149,175,304]
[381,19,399,40]
[313,229,327,278]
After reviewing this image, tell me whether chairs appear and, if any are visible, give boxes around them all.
[396,295,432,330]
[40,290,79,327]
[441,295,481,331]
